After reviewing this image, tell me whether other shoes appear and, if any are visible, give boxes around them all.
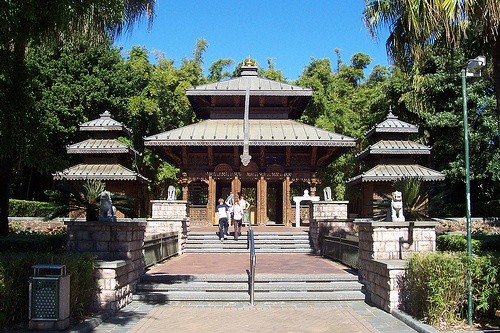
[238,233,241,236]
[224,235,227,238]
[221,238,224,242]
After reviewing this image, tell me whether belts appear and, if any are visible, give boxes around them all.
[243,209,245,210]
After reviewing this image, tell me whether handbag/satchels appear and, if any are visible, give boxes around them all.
[231,212,234,217]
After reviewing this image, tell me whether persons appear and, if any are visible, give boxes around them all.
[217,192,250,242]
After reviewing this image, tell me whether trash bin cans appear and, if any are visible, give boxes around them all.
[29,265,70,321]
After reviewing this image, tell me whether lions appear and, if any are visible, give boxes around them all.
[387,192,403,218]
[99,190,115,218]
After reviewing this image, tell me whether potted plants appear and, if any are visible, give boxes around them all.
[42,180,138,222]
